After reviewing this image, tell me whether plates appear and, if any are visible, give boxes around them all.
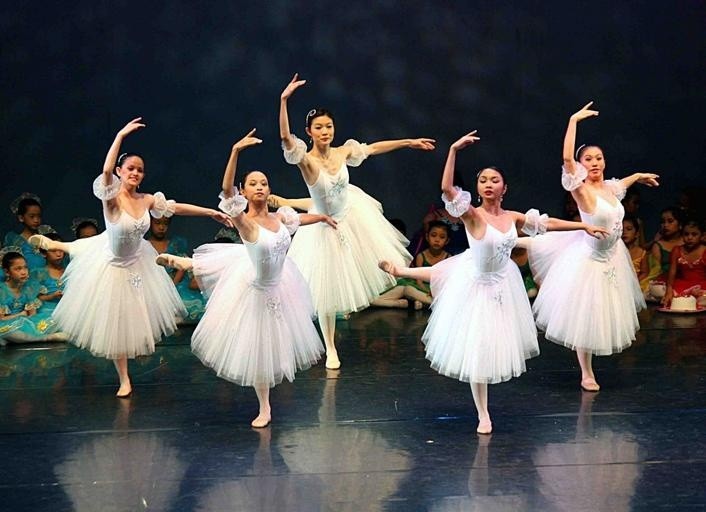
[657,305,706,315]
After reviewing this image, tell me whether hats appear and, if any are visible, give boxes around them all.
[656,294,706,314]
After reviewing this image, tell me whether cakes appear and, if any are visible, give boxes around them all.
[670,294,697,310]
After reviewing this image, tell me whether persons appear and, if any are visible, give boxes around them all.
[337,196,469,320]
[621,190,705,313]
[280,73,438,370]
[379,128,609,434]
[564,191,582,222]
[1,192,103,345]
[516,101,661,392]
[510,247,545,334]
[29,116,235,397]
[145,210,244,325]
[156,127,337,428]
